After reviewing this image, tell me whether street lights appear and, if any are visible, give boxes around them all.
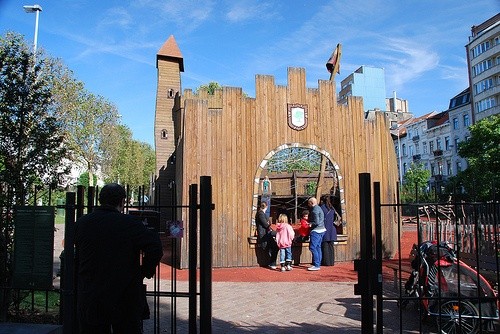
[22,4,43,205]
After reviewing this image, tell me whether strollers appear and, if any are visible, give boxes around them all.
[397,240,500,334]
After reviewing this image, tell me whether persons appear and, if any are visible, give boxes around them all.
[320,195,337,266]
[276,214,296,272]
[307,197,327,271]
[74,183,164,334]
[255,201,279,270]
[276,202,291,224]
[295,210,312,243]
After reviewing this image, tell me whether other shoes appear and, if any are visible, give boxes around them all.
[268,264,280,270]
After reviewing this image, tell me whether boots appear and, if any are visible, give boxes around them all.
[280,261,293,271]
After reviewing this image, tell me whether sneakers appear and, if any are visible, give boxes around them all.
[308,266,320,270]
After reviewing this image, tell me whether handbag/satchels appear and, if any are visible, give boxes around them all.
[334,215,342,226]
